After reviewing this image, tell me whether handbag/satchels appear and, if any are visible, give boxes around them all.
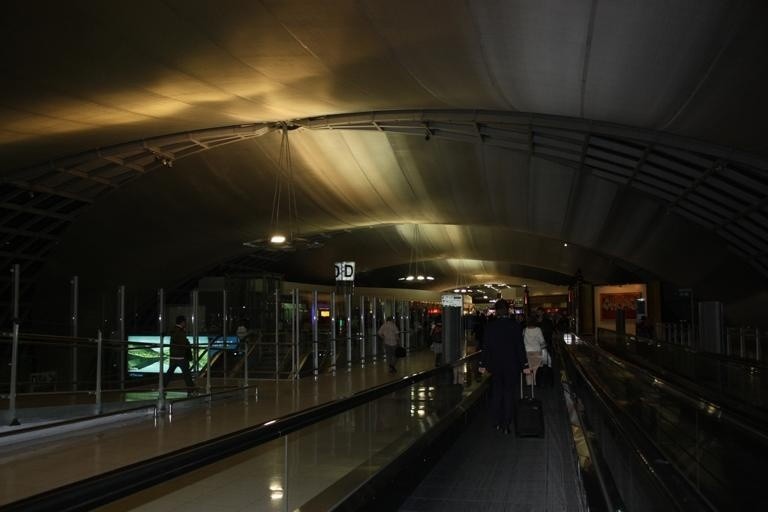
[393,344,407,359]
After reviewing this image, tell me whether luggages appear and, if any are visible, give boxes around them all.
[535,345,555,390]
[514,366,545,440]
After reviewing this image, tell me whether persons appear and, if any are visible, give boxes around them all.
[163,316,194,388]
[378,316,402,374]
[430,318,442,366]
[475,298,554,435]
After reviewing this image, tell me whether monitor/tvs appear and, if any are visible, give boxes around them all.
[441,294,462,307]
